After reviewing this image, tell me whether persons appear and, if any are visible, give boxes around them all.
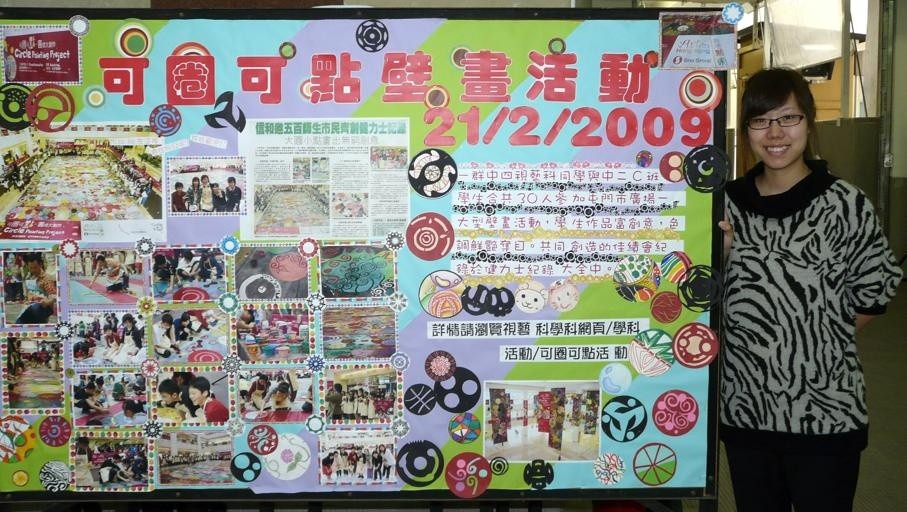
[717,66,905,511]
[153,250,224,293]
[89,253,130,293]
[5,253,56,323]
[172,175,241,211]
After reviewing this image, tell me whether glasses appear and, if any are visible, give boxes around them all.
[746,114,807,130]
